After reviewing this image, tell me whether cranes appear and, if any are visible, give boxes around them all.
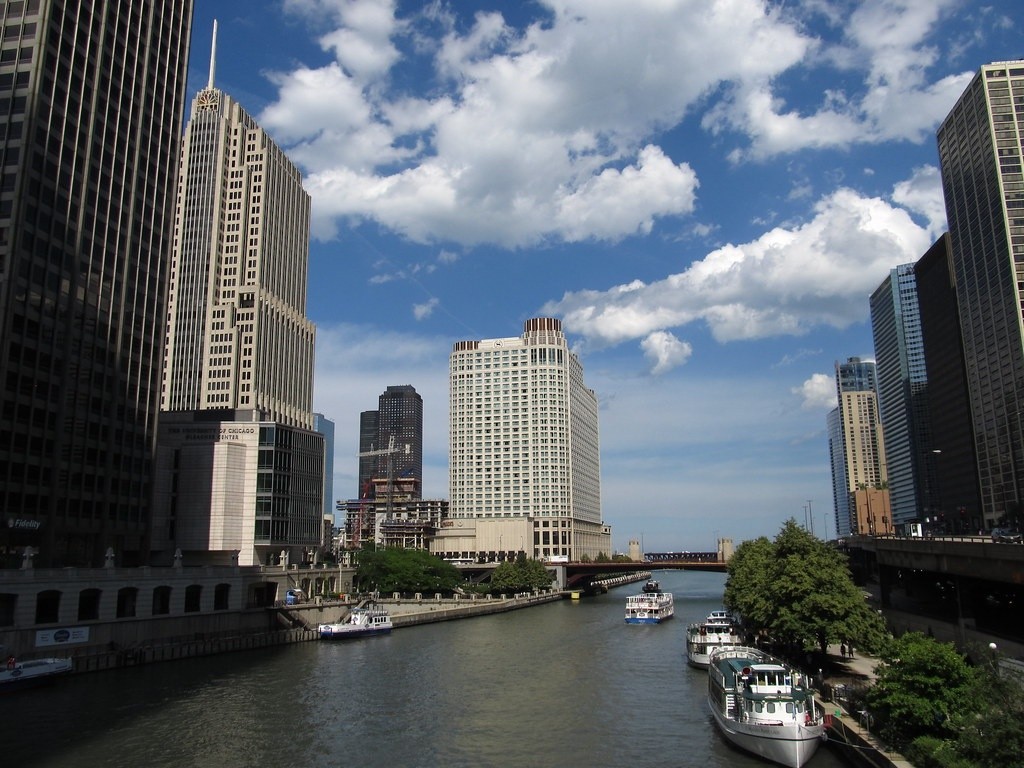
[356,435,404,521]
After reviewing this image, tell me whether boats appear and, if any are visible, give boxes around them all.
[317,604,393,639]
[686,623,740,668]
[0,656,73,684]
[625,579,674,624]
[707,645,824,768]
[705,609,738,624]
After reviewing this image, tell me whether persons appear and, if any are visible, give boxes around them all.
[848,643,854,658]
[841,643,846,657]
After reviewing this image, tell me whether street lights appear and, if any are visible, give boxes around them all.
[921,449,943,533]
[880,463,890,536]
[338,562,345,593]
[824,513,830,541]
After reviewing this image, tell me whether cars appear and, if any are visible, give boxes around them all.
[991,528,1022,544]
